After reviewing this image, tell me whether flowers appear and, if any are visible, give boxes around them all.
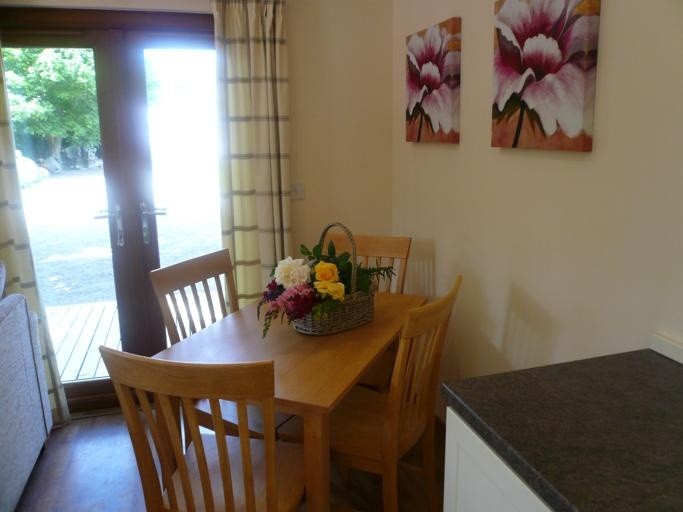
[253,238,398,338]
[401,18,460,144]
[490,0,601,150]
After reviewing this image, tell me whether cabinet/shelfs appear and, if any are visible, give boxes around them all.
[438,345,683,511]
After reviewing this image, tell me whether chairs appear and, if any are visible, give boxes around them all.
[271,273,466,512]
[148,247,297,458]
[0,257,54,512]
[97,342,308,510]
[315,228,413,397]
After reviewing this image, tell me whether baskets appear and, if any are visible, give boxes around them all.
[291,222,374,336]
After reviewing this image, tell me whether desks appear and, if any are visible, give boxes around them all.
[146,281,430,511]
